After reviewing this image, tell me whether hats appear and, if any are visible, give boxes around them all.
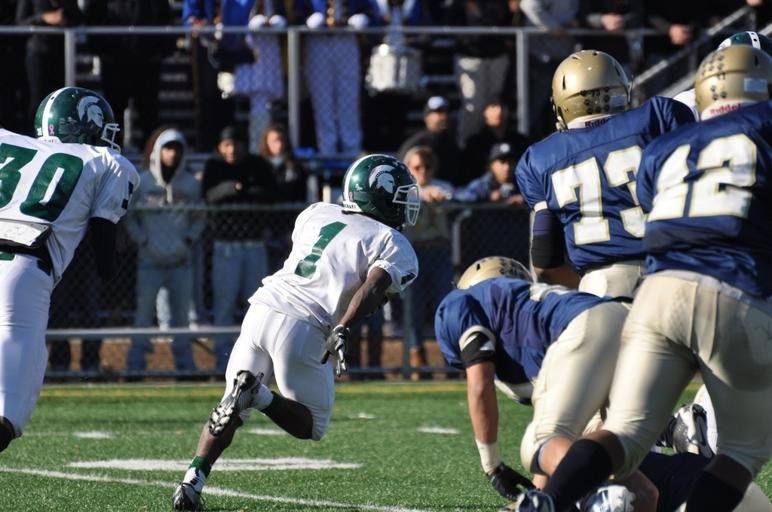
[423,95,452,116]
[487,143,517,164]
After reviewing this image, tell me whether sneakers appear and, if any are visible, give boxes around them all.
[515,489,556,512]
[172,482,206,511]
[670,403,715,460]
[580,484,636,511]
[209,369,261,435]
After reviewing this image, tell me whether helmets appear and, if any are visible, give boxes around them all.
[694,44,772,123]
[340,153,422,232]
[715,30,771,59]
[549,49,631,131]
[456,255,533,291]
[33,85,123,152]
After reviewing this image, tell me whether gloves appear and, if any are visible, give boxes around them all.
[485,461,542,502]
[320,325,352,376]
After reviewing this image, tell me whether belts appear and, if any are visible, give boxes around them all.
[0,242,53,268]
